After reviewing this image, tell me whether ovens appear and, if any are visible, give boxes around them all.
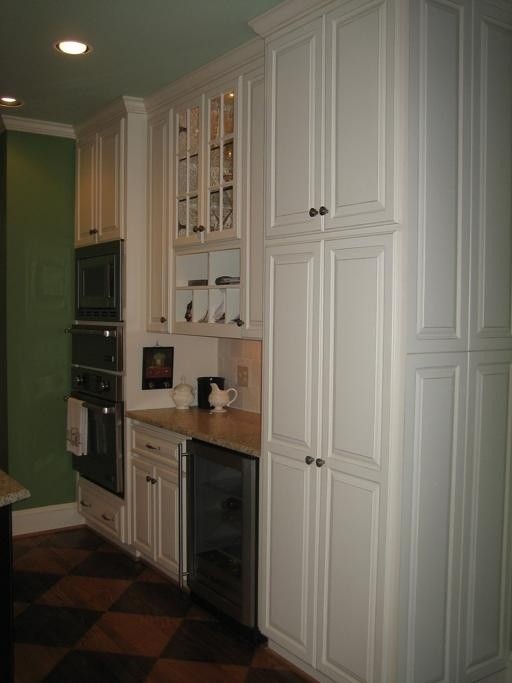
[65,391,124,495]
[69,325,123,403]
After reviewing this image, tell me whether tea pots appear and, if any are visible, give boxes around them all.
[208,382,238,413]
[168,377,196,410]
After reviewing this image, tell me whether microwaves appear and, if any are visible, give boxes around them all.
[76,240,122,322]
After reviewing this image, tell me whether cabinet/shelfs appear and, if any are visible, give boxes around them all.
[77,476,125,544]
[175,439,257,631]
[73,117,126,244]
[256,1,509,680]
[147,53,263,342]
[129,421,187,582]
